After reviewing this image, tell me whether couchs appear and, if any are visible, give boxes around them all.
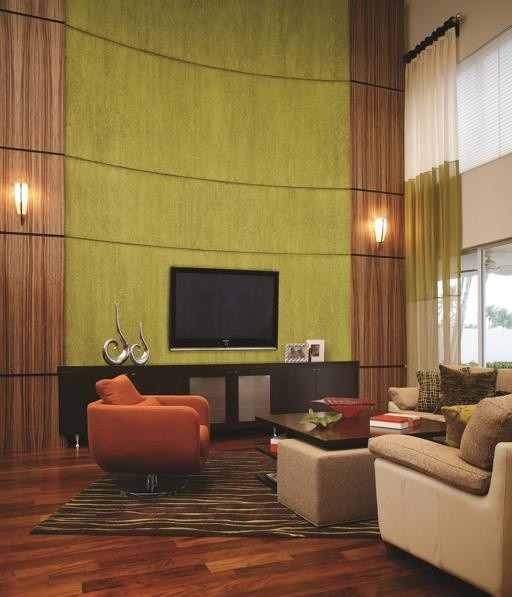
[386,364,511,431]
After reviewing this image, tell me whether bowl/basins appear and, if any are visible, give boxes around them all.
[305,397,378,418]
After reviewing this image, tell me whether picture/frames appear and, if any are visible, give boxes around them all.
[284,338,325,364]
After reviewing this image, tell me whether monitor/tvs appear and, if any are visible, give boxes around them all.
[169,265,279,351]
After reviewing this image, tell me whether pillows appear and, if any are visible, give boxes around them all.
[415,359,498,447]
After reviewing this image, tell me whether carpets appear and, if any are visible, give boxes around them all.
[29,446,382,539]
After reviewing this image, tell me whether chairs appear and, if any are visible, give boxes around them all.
[86,371,211,496]
[366,392,512,596]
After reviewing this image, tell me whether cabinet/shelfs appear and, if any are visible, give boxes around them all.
[56,359,360,450]
